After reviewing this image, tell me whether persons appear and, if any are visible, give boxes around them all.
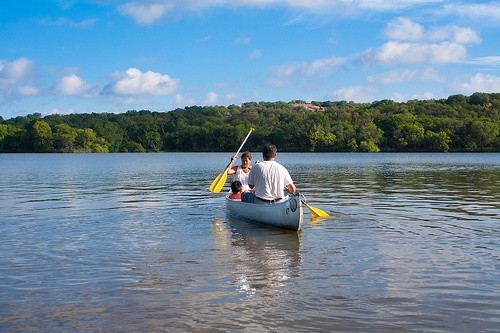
[228,181,244,200]
[242,144,296,205]
[227,152,253,197]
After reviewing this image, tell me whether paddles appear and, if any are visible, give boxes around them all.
[210,128,255,193]
[284,186,330,217]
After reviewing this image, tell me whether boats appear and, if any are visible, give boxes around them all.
[225,183,303,232]
